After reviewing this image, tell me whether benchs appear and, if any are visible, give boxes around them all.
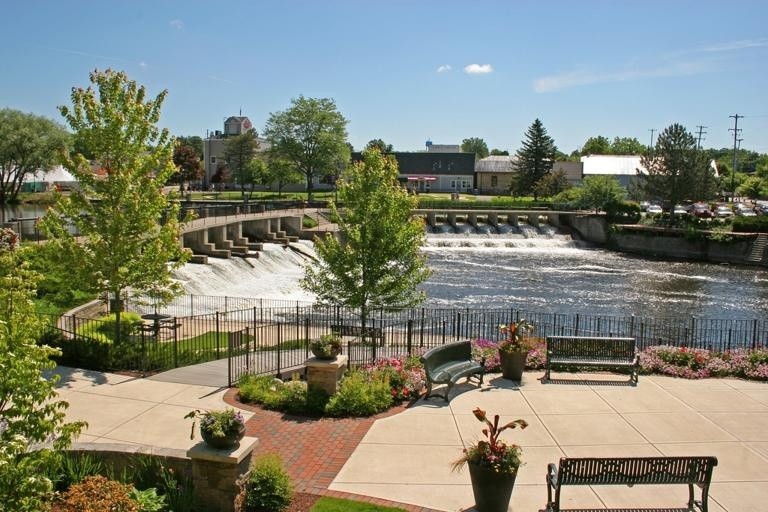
[545,455,718,512]
[545,336,640,386]
[419,340,484,402]
[331,325,385,346]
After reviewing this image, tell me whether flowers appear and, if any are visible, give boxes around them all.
[184,405,246,440]
[501,318,534,352]
[451,406,528,477]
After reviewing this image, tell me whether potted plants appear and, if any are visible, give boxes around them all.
[309,331,343,360]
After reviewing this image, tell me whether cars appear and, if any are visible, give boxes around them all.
[639,200,768,218]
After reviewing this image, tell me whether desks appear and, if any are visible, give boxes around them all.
[141,313,171,336]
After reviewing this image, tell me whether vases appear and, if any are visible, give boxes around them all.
[200,425,246,449]
[468,462,519,512]
[499,348,527,380]
